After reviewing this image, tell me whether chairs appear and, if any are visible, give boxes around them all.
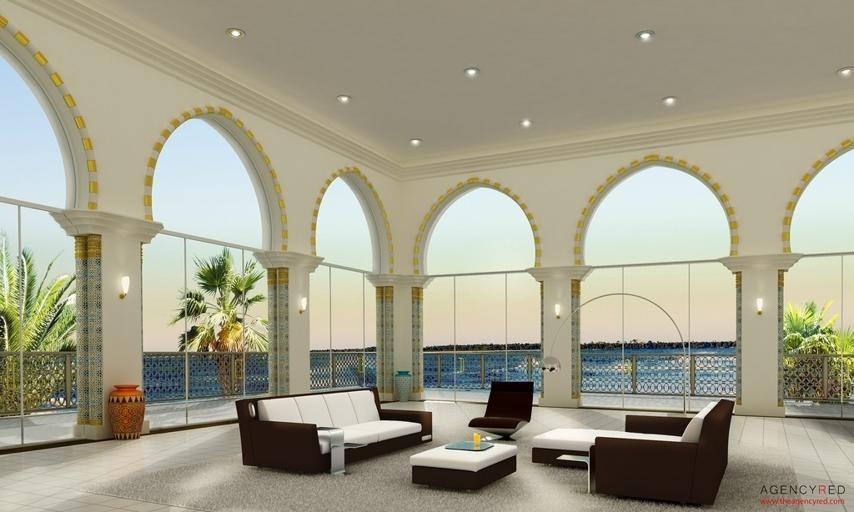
[468,380,536,440]
[531,400,736,506]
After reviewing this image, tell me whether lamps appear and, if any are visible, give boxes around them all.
[754,297,764,315]
[554,302,561,319]
[540,291,686,417]
[118,274,131,300]
[298,297,308,314]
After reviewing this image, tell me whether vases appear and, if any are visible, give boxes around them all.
[394,370,412,402]
[108,381,146,439]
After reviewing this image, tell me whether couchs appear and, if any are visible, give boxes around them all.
[235,387,433,474]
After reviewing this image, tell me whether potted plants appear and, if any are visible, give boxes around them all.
[168,246,269,397]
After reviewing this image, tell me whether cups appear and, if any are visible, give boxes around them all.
[473,432,481,446]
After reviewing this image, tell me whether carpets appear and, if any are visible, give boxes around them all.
[76,436,803,512]
